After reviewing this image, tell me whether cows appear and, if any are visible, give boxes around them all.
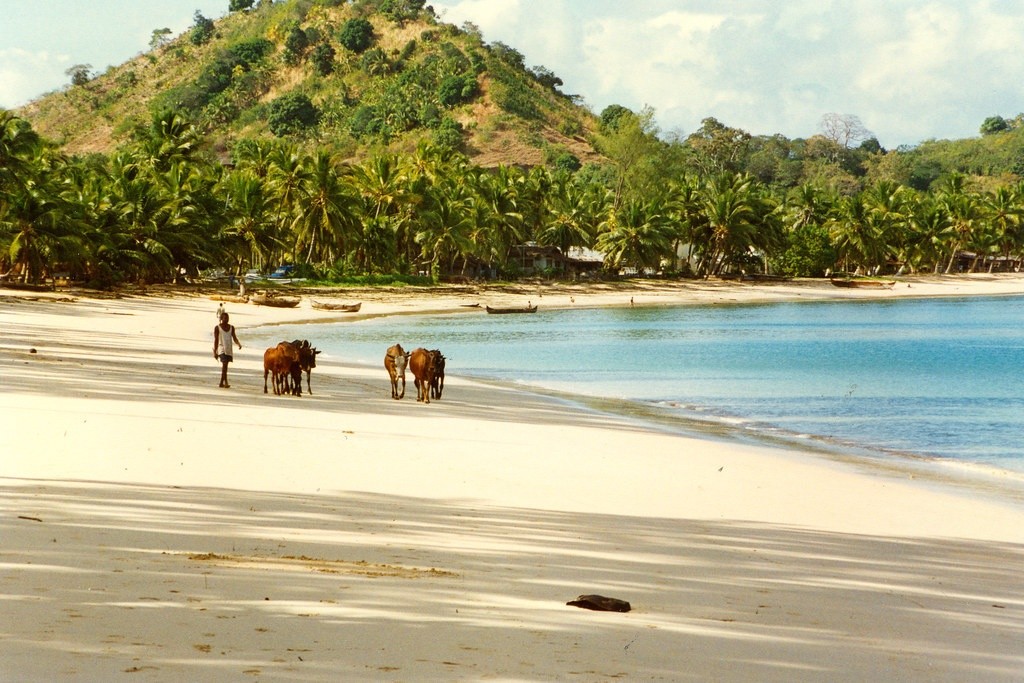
[264,340,322,397]
[385,344,446,404]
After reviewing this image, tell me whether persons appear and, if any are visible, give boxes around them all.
[213,312,243,388]
[239,274,246,296]
[216,302,225,324]
[229,273,235,289]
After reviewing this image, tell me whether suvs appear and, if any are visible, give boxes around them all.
[246,269,263,277]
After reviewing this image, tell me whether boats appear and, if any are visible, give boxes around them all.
[486,306,538,312]
[253,294,299,308]
[829,275,897,288]
[210,293,248,303]
[309,299,361,312]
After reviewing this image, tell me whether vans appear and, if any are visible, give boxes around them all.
[272,266,300,278]
